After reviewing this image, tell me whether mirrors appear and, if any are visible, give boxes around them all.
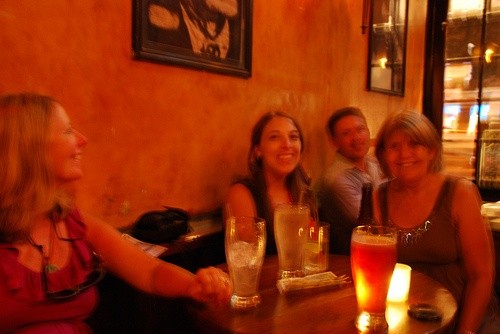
[367,0,410,95]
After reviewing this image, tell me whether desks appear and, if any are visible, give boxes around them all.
[188,253,458,333]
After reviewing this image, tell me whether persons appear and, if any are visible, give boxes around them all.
[0,93,233,334]
[310,107,387,254]
[372,109,494,334]
[223,110,324,260]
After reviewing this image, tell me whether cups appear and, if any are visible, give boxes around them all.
[305,222,330,275]
[273,202,309,279]
[350,226,397,334]
[225,217,267,309]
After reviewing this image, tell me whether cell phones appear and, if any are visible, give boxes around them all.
[408,303,440,320]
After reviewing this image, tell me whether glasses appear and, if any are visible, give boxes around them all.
[37,240,106,300]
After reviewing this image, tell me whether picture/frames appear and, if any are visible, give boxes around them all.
[131,0,254,80]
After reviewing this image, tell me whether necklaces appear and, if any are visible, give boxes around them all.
[25,217,59,273]
